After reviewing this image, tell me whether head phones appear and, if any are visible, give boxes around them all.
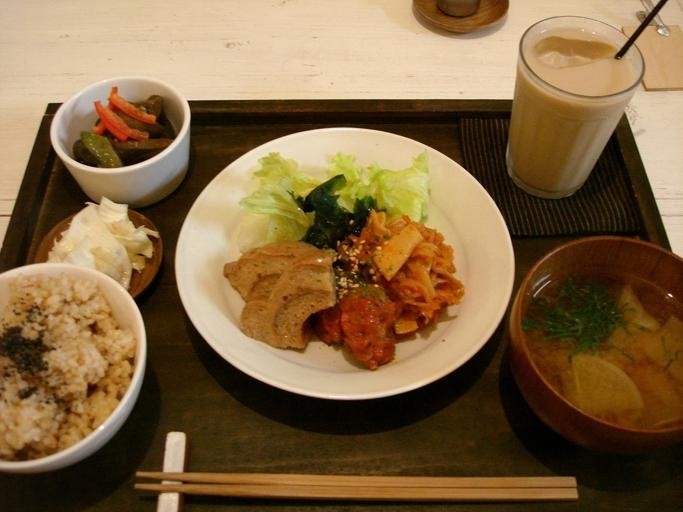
[135,470,577,500]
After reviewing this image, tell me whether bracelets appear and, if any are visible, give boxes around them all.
[510,237,683,448]
[50,77,192,207]
[0,262,147,475]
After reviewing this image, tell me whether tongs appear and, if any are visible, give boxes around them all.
[0,273,136,460]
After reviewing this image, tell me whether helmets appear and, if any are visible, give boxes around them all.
[34,207,162,299]
[174,126,514,401]
[411,1,512,36]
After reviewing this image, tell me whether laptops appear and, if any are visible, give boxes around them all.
[223,240,337,351]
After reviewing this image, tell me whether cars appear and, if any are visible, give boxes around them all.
[507,14,644,198]
